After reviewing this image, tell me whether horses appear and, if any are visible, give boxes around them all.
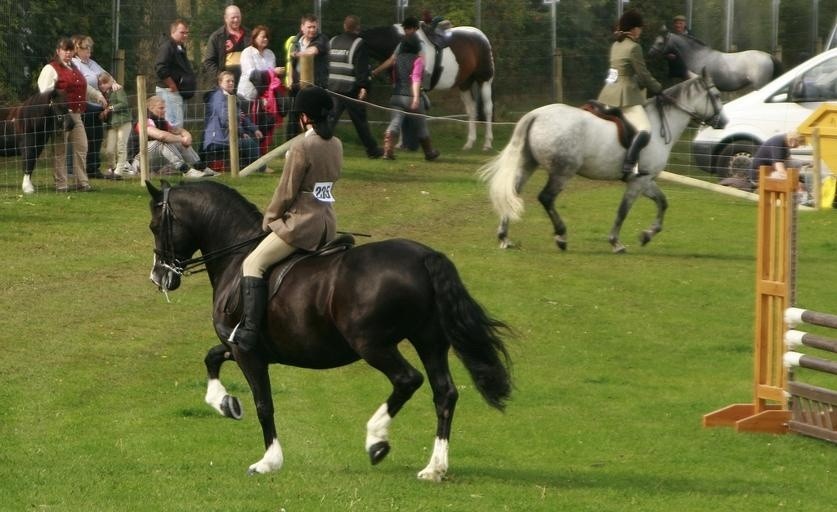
[0,80,77,196]
[645,24,780,94]
[347,15,498,153]
[471,66,729,255]
[144,178,520,482]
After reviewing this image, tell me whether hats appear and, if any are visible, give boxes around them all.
[618,10,644,32]
[400,15,420,31]
[293,86,334,116]
[673,15,688,20]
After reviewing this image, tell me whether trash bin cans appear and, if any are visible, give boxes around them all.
[797,102,837,209]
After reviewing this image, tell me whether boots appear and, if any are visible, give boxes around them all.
[620,130,651,176]
[382,130,397,160]
[215,275,268,352]
[419,136,440,162]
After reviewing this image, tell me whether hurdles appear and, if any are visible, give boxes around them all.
[704,166,837,443]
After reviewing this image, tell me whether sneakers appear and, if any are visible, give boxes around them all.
[182,167,222,178]
[88,169,124,181]
[55,182,96,194]
[368,147,386,160]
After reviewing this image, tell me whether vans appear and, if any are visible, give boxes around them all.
[688,46,837,194]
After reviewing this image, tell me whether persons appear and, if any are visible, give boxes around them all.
[597,9,664,176]
[750,130,804,192]
[664,13,707,86]
[215,84,344,352]
[22,3,439,195]
